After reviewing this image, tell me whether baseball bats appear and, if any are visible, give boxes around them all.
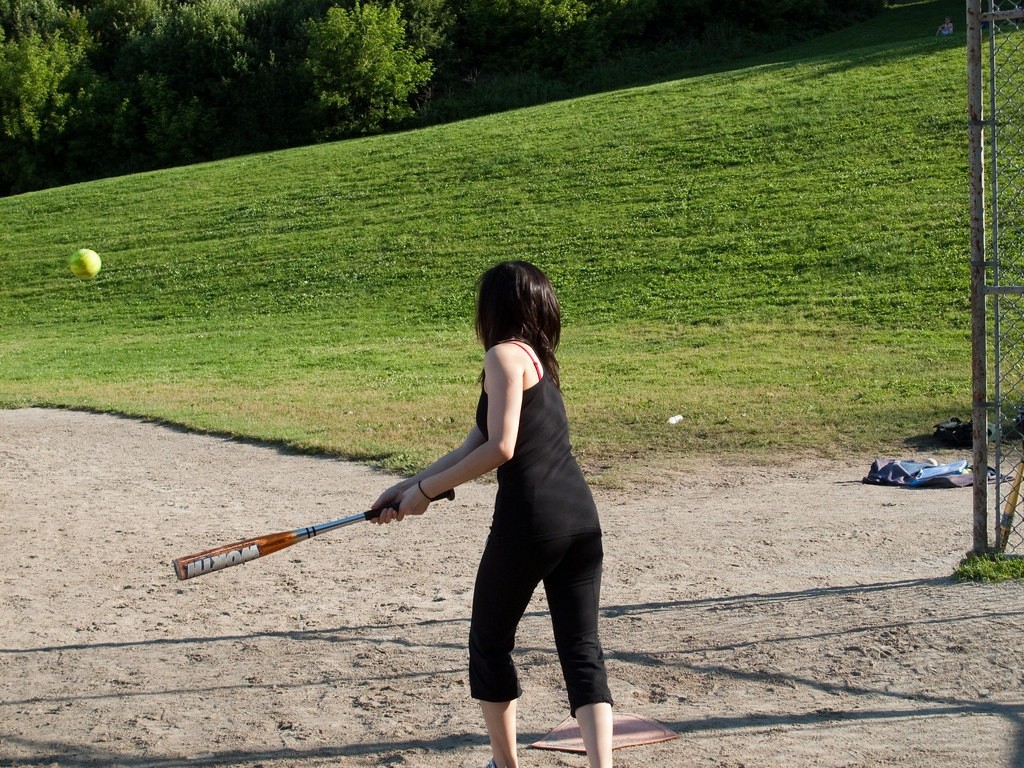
[173,487,456,581]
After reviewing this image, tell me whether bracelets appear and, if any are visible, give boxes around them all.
[418,481,432,500]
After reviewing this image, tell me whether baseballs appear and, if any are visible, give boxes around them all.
[70,248,102,280]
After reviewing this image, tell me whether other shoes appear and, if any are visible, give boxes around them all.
[486,758,498,768]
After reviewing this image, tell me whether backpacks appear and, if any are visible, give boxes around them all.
[935,415,991,446]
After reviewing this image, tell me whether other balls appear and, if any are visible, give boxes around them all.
[668,415,684,425]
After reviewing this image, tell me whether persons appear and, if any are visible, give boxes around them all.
[371,261,614,768]
